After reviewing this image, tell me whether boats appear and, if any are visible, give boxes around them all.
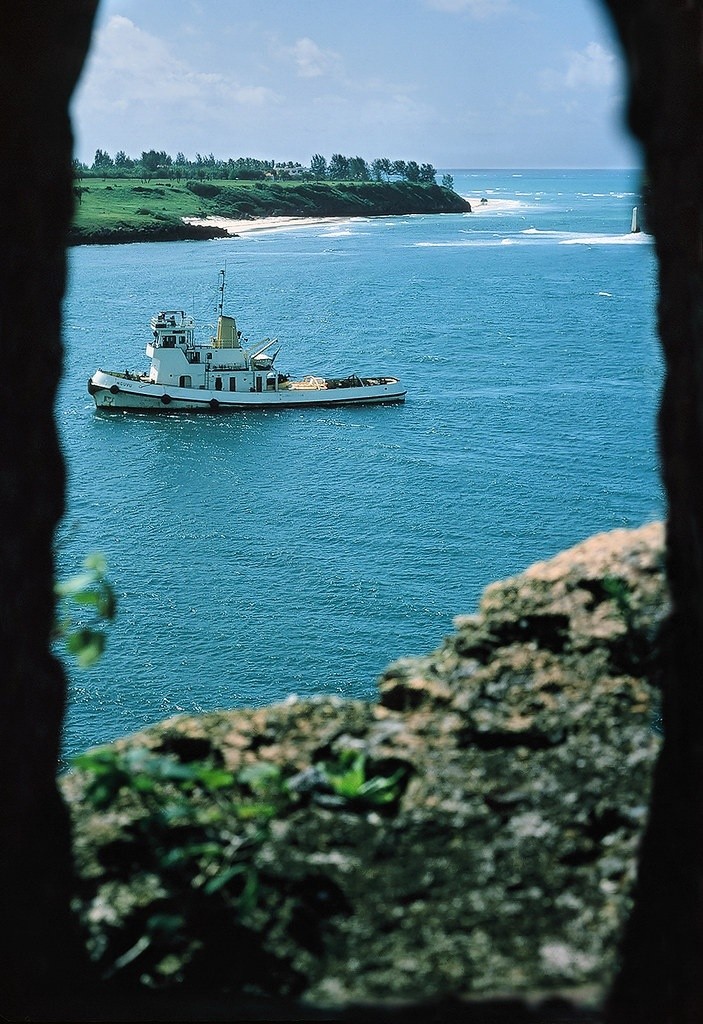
[88,259,408,412]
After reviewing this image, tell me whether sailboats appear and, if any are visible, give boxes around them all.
[630,207,641,233]
